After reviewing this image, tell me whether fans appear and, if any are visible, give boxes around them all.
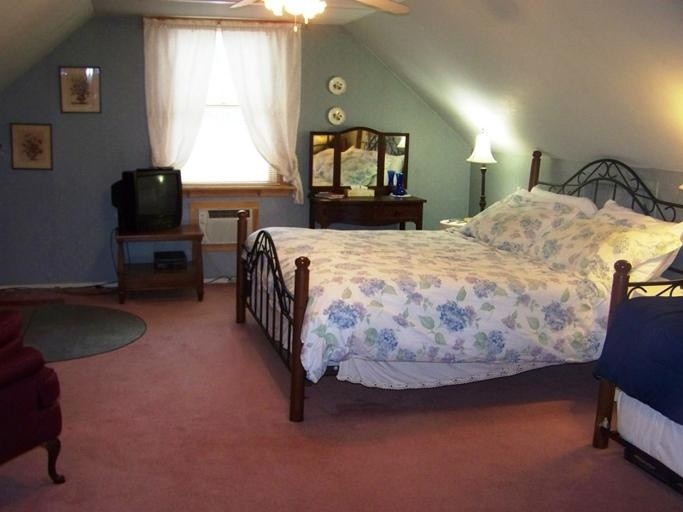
[230,0,410,16]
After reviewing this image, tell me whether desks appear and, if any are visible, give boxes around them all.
[308,193,427,229]
[113,226,214,304]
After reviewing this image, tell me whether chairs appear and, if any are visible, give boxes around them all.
[1,307,66,484]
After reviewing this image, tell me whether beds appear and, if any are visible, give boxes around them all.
[236,151,683,422]
[592,260,683,499]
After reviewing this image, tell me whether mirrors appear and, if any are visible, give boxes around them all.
[308,125,409,195]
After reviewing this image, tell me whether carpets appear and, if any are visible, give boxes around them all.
[1,302,147,364]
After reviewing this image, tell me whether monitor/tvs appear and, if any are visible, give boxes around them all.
[110,169,182,234]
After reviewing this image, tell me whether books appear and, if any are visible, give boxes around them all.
[315,194,343,200]
[318,191,345,197]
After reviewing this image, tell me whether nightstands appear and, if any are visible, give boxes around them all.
[440,210,473,229]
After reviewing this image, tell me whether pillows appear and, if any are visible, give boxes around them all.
[459,184,683,294]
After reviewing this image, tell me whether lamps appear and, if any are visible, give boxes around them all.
[467,133,498,211]
[264,0,327,33]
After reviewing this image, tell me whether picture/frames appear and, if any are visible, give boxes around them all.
[59,66,102,113]
[9,121,53,171]
